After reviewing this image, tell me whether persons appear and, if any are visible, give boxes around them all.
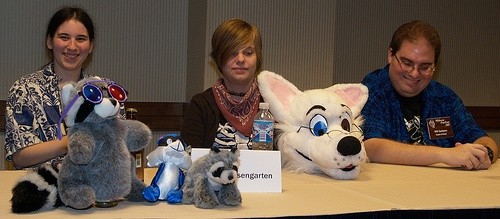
[4,8,96,168]
[361,21,498,170]
[180,19,278,150]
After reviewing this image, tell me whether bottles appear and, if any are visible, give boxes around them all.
[252,103,274,150]
[126,108,144,183]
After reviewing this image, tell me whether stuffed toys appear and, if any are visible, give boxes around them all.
[257,71,369,180]
[142,134,192,204]
[183,147,242,208]
[12,77,153,213]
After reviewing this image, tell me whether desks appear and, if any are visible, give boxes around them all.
[0,157,500,219]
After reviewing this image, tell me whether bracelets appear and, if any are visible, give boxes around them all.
[486,146,493,162]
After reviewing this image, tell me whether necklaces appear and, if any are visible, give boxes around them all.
[230,91,245,97]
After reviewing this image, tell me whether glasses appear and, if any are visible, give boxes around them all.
[58,80,128,141]
[392,50,436,75]
